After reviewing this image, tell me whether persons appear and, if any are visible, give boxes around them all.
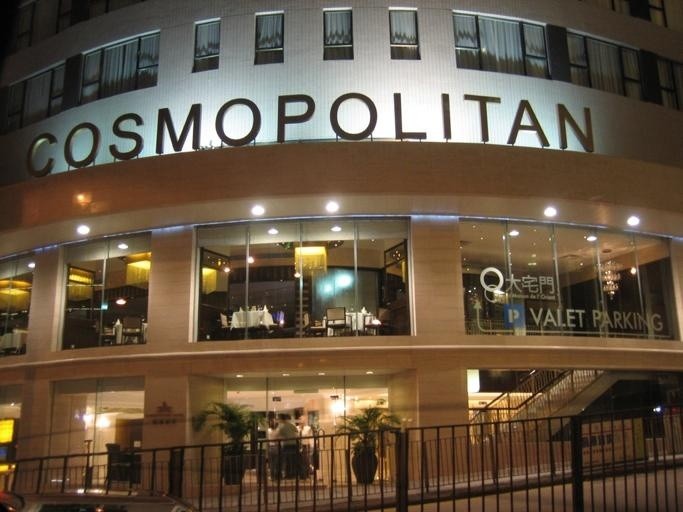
[295,415,315,480]
[267,418,280,481]
[275,413,299,480]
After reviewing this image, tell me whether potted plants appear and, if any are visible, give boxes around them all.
[332,406,405,484]
[189,399,267,485]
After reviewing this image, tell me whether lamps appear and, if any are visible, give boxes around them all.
[291,242,329,276]
[125,251,152,290]
[598,249,622,301]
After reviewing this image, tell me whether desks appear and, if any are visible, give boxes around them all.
[2,331,27,354]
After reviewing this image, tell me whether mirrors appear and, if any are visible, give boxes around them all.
[199,245,231,315]
[67,266,95,320]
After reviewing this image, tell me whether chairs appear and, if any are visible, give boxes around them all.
[105,442,136,495]
[268,310,300,337]
[303,307,379,337]
[218,312,231,338]
